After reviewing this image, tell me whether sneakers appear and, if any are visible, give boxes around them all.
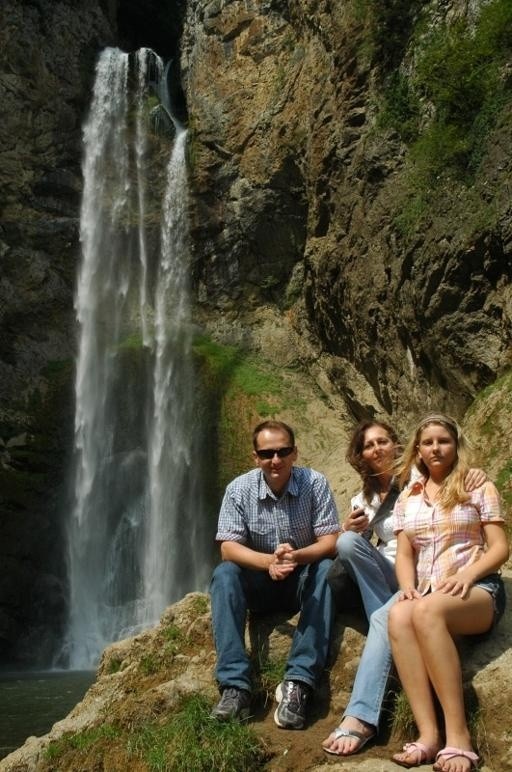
[274,680,311,730]
[208,688,252,723]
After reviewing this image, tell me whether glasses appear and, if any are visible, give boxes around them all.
[255,446,294,459]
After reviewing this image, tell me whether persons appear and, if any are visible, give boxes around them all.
[207,421,339,732]
[321,421,489,757]
[389,413,510,772]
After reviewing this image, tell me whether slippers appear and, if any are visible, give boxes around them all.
[321,727,484,772]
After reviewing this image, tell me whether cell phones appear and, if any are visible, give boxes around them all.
[354,505,364,518]
